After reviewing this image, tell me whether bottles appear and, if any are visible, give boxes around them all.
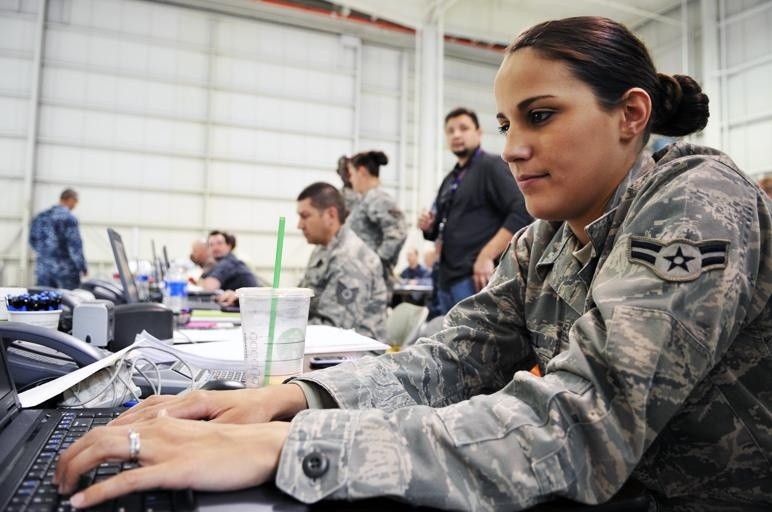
[163,257,189,315]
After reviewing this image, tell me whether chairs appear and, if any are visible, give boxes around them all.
[387,301,446,349]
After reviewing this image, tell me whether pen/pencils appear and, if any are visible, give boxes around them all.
[6,288,63,311]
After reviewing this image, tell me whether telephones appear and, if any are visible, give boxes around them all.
[0,320,117,385]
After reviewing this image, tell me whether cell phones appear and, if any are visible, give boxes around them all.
[310,354,358,370]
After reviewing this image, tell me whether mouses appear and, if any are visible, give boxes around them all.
[201,378,247,390]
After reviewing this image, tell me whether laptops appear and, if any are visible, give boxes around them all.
[1,349,201,510]
[108,228,222,344]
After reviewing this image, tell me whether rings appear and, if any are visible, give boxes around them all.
[126,429,141,462]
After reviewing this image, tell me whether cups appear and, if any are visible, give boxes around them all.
[235,286,316,389]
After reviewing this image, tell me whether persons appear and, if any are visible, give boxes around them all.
[28,188,89,291]
[190,239,216,273]
[343,149,411,304]
[48,15,772,512]
[415,106,529,315]
[213,182,390,358]
[400,247,428,279]
[197,230,258,308]
[336,154,364,214]
